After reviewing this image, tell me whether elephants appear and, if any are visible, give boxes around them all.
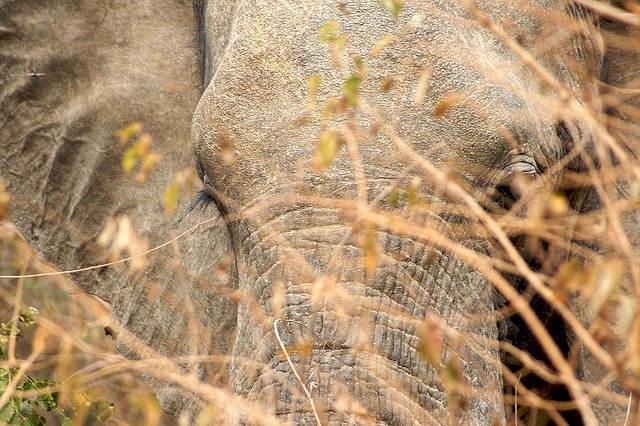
[1,0,639,426]
